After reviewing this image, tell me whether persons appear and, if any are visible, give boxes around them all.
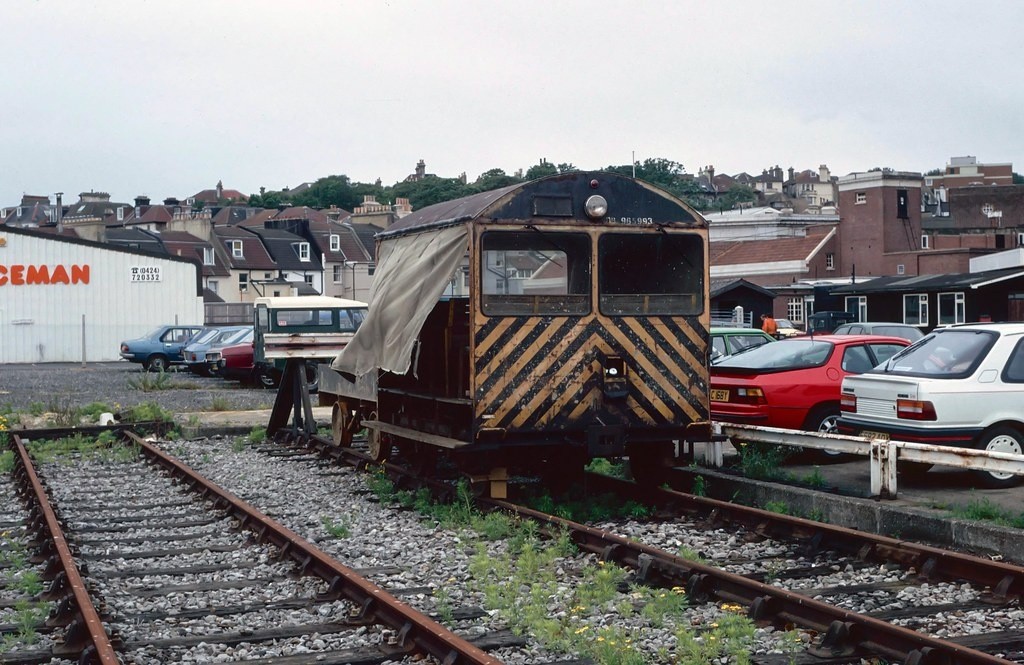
[761,314,778,337]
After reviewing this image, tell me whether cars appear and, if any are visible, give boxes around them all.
[710,312,953,364]
[184,297,371,393]
[119,326,206,372]
[710,335,964,463]
[836,323,1024,488]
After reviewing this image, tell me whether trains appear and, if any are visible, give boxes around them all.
[316,172,729,500]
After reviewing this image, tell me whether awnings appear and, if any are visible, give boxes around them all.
[827,264,1024,296]
